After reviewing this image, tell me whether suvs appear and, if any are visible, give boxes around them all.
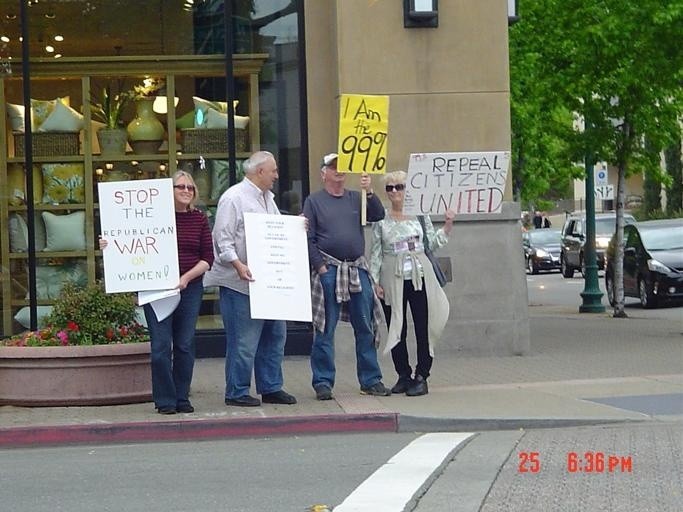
[561,210,634,277]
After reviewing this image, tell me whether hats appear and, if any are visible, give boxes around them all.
[321,153,338,168]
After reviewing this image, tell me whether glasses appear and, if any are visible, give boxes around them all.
[385,184,406,192]
[323,164,338,170]
[174,184,195,192]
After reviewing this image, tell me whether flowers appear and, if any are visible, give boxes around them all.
[1,316,150,346]
[126,77,167,101]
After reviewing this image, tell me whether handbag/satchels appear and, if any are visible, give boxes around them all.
[416,216,447,287]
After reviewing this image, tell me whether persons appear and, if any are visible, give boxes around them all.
[97,169,215,415]
[198,150,312,408]
[300,149,393,401]
[364,170,457,397]
[519,210,551,231]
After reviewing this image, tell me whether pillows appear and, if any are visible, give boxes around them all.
[8,161,88,300]
[203,108,249,129]
[39,98,89,133]
[30,94,71,133]
[192,96,239,128]
[13,305,56,331]
[179,160,248,230]
[6,102,34,134]
[175,110,196,131]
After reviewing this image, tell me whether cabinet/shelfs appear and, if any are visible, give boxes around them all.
[1,53,269,335]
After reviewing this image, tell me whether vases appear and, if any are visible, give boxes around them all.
[126,95,165,155]
[96,170,131,183]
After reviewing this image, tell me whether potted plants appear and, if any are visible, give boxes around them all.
[0,276,163,405]
[88,77,129,154]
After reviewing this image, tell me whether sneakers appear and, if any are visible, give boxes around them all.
[316,385,331,400]
[360,382,392,396]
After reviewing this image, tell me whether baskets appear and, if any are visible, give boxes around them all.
[180,128,249,154]
[12,130,80,156]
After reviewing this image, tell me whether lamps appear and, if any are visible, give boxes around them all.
[152,0,180,116]
[507,0,520,27]
[401,0,440,28]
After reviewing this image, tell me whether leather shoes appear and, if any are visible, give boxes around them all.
[406,376,427,396]
[178,405,194,412]
[158,408,177,414]
[225,395,261,406]
[391,374,411,393]
[263,390,297,403]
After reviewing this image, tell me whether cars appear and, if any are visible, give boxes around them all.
[522,229,561,274]
[605,218,682,305]
